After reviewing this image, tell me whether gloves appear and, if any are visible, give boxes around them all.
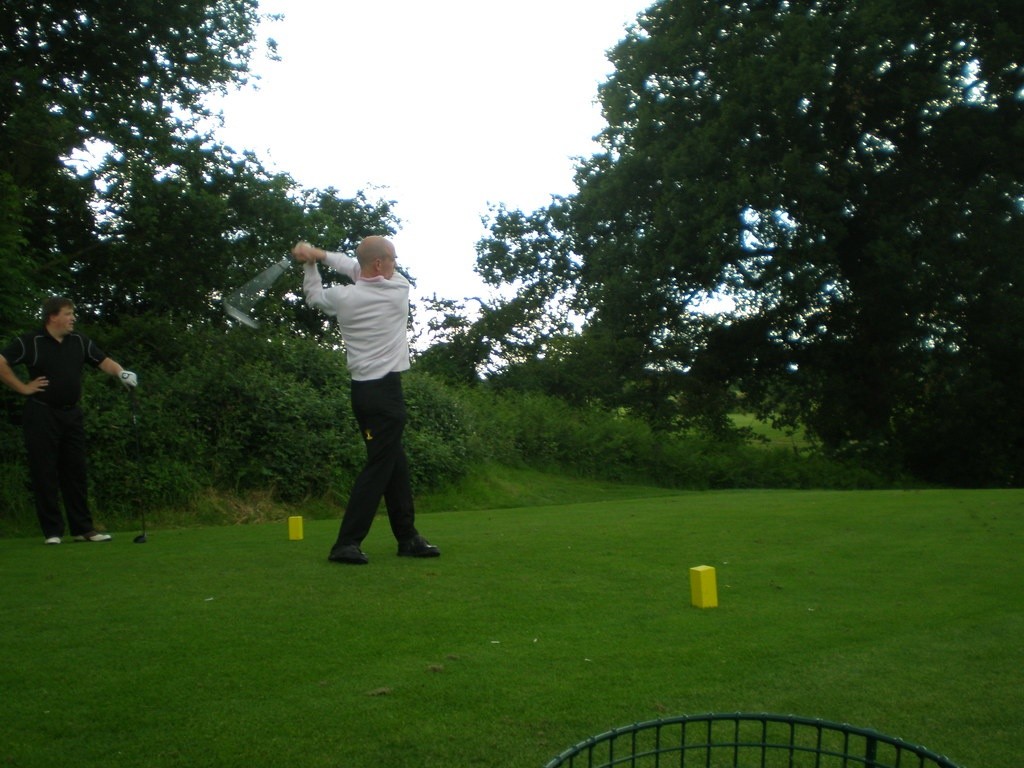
[118,369,138,391]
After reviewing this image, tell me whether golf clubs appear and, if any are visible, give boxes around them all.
[222,257,302,326]
[128,388,147,540]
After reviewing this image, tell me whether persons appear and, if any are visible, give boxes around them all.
[291,235,441,564]
[0,297,138,545]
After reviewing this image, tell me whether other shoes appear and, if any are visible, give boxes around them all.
[44,536,62,545]
[328,550,368,565]
[396,540,440,558]
[74,530,111,542]
